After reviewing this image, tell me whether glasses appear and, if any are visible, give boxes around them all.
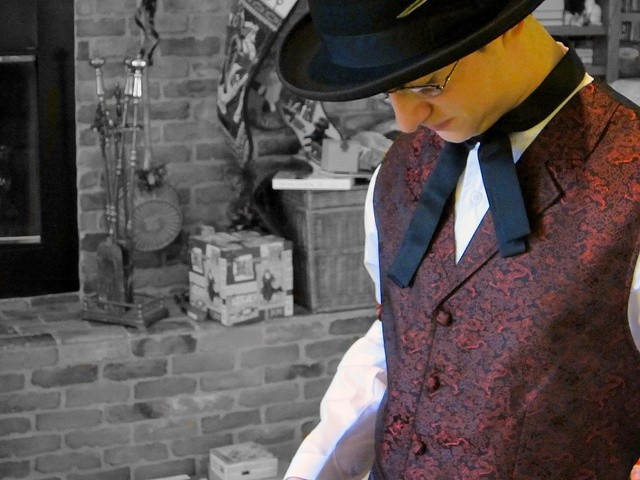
[366,55,462,102]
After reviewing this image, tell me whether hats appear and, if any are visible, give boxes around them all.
[277,0,548,103]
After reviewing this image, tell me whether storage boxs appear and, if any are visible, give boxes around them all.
[276,185,379,314]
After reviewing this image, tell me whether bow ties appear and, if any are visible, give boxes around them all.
[388,47,585,288]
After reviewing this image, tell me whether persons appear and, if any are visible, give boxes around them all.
[276,0,640,480]
